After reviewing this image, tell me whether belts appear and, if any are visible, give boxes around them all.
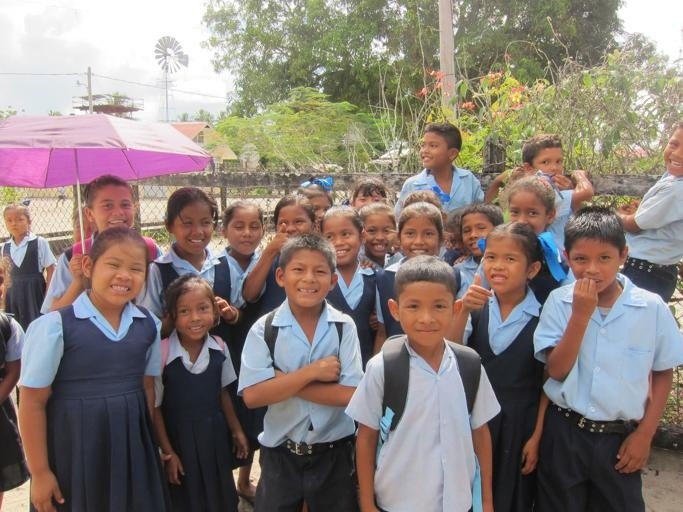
[551,400,628,434]
[625,256,678,283]
[285,433,354,456]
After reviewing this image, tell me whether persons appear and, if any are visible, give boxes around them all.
[2,119,683,512]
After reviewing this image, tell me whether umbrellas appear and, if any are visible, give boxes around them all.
[0,115,213,255]
